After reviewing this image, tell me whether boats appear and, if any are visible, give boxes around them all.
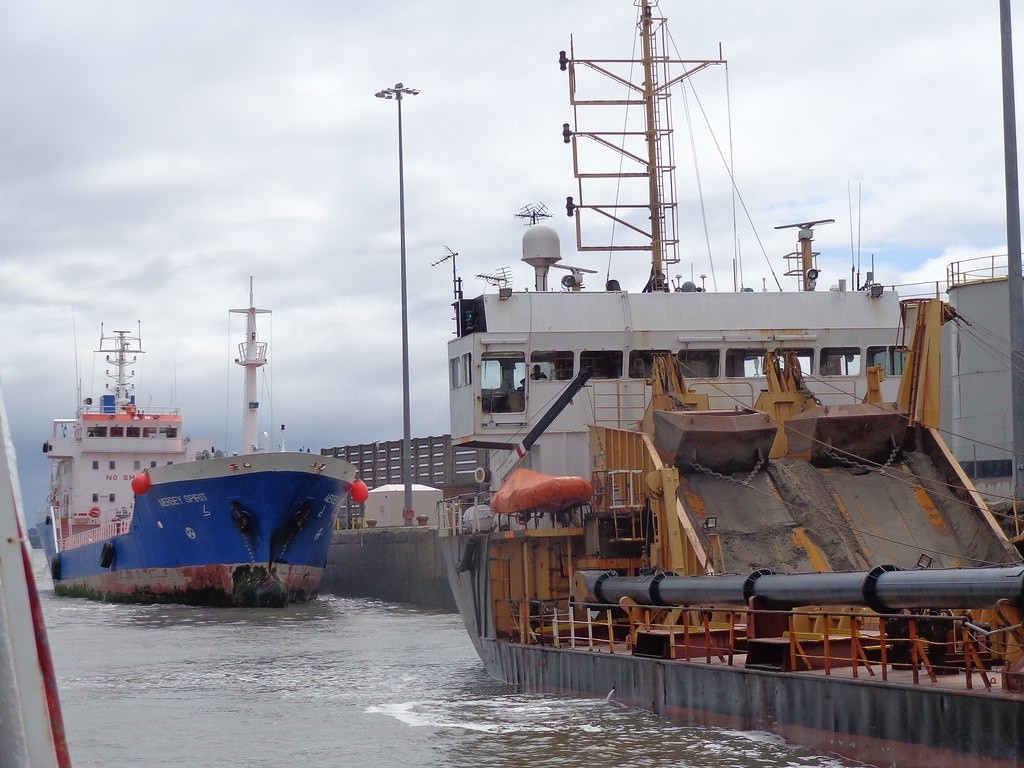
[435,1,1024,768]
[490,469,593,515]
[35,275,358,609]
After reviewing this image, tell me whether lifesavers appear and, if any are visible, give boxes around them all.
[99,541,114,569]
[51,557,61,579]
[403,508,416,520]
[90,506,101,517]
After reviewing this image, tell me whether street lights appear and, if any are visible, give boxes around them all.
[374,83,421,526]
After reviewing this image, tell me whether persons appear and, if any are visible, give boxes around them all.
[530,364,547,381]
